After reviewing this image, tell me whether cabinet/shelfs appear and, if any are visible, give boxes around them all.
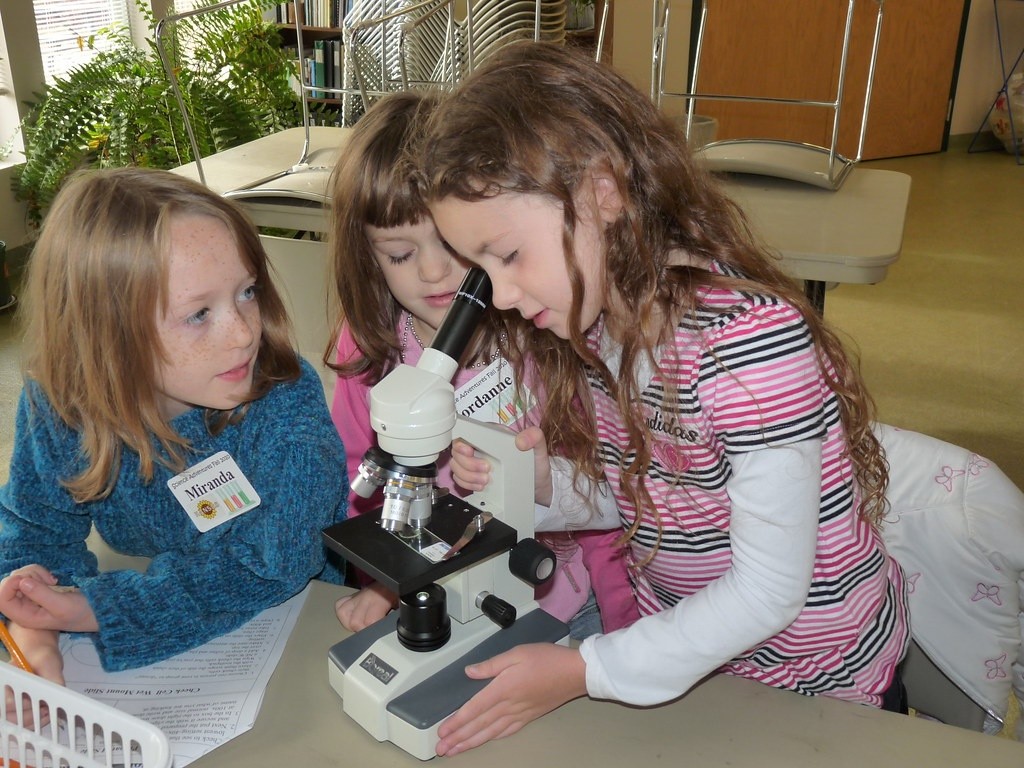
[278,0,349,127]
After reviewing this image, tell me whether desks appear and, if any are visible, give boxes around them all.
[76,582,1024,768]
[156,125,911,325]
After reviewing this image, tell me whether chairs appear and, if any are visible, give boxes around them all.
[869,419,1024,731]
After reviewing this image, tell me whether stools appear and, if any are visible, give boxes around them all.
[152,0,879,222]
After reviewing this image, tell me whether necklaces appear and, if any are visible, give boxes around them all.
[400,313,505,372]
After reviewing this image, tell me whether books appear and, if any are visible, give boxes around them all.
[275,0,359,128]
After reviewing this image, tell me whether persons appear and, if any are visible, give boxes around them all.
[324,89,591,631]
[0,165,348,732]
[411,39,910,756]
